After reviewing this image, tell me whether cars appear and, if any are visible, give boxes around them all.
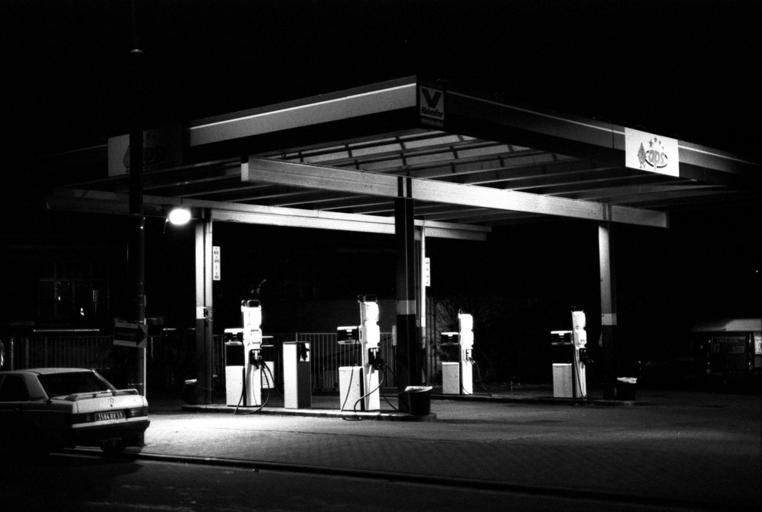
[0,367,151,461]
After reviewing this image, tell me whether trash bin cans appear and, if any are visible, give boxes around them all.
[408,389,431,416]
[616,377,637,400]
